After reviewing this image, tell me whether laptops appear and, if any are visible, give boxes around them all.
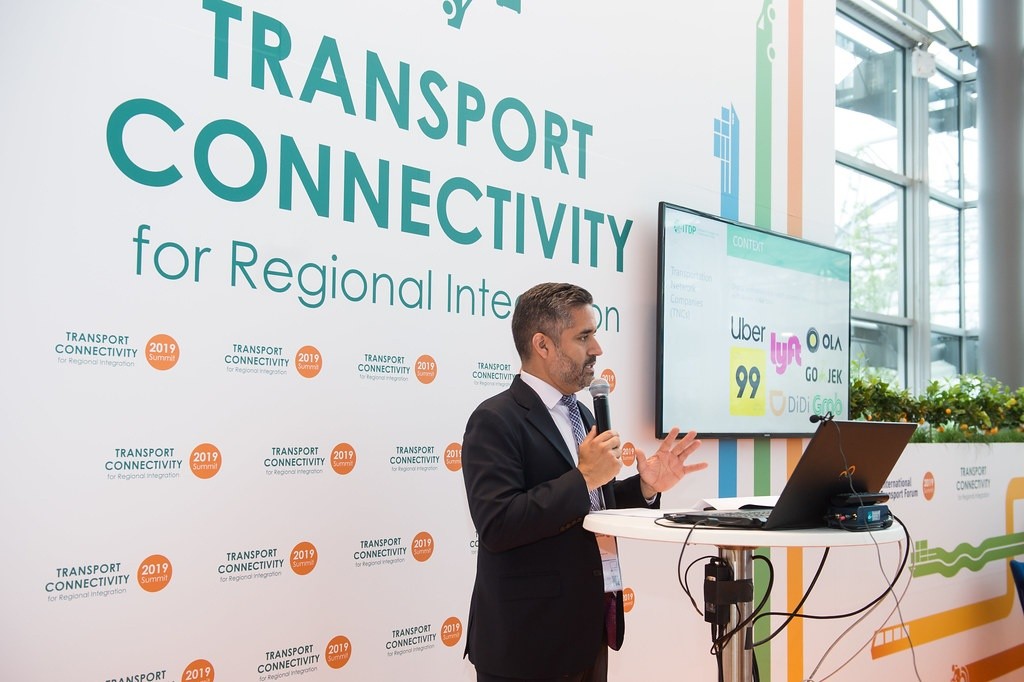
[662,422,919,529]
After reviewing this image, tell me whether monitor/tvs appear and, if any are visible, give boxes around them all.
[653,200,852,441]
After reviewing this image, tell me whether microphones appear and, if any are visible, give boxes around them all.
[808,411,832,423]
[589,378,616,487]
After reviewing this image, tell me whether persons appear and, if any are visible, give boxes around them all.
[461,282,708,682]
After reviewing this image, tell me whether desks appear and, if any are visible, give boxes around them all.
[582,508,906,682]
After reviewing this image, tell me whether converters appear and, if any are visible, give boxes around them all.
[704,563,730,624]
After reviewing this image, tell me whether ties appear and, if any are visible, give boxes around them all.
[561,391,621,598]
